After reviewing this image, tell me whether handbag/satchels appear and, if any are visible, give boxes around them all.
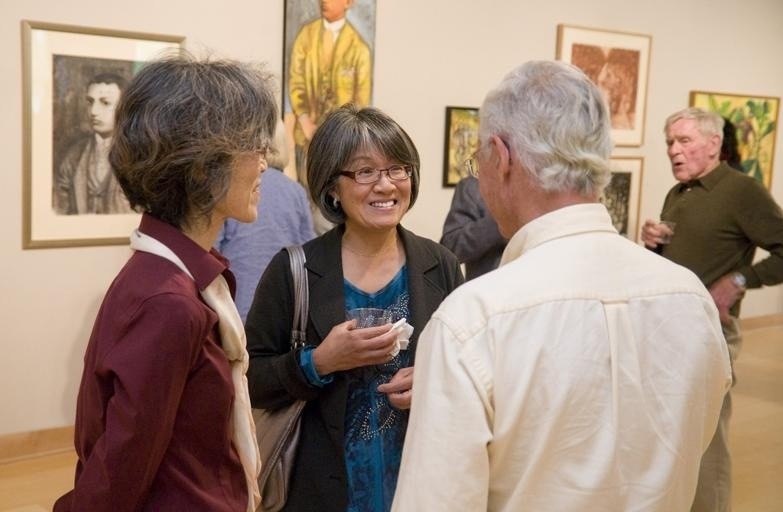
[250,246,309,512]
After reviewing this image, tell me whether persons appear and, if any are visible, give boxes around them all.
[212,123,315,510]
[243,102,467,511]
[392,59,733,512]
[53,45,278,512]
[641,108,782,511]
[289,1,374,201]
[439,167,511,281]
[53,73,143,213]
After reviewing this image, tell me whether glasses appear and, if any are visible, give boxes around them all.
[466,140,512,179]
[340,164,414,183]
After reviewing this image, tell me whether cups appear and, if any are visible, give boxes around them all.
[657,220,677,245]
[343,307,394,330]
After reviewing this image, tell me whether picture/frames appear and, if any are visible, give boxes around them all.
[689,90,781,190]
[21,18,187,250]
[282,0,376,237]
[442,106,480,188]
[600,156,646,244]
[556,23,654,148]
